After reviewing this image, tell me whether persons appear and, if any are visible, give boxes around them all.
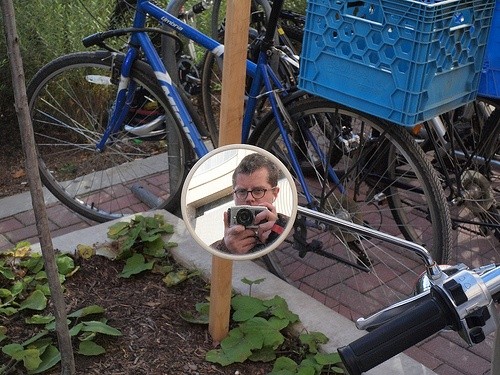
[207,153,290,256]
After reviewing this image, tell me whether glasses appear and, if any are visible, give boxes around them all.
[233,188,273,199]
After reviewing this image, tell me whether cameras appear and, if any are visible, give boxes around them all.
[227,205,268,228]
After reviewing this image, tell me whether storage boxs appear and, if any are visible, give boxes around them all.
[296,0,495,126]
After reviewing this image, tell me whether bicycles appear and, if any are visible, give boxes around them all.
[201,0,500,301]
[25,0,451,336]
